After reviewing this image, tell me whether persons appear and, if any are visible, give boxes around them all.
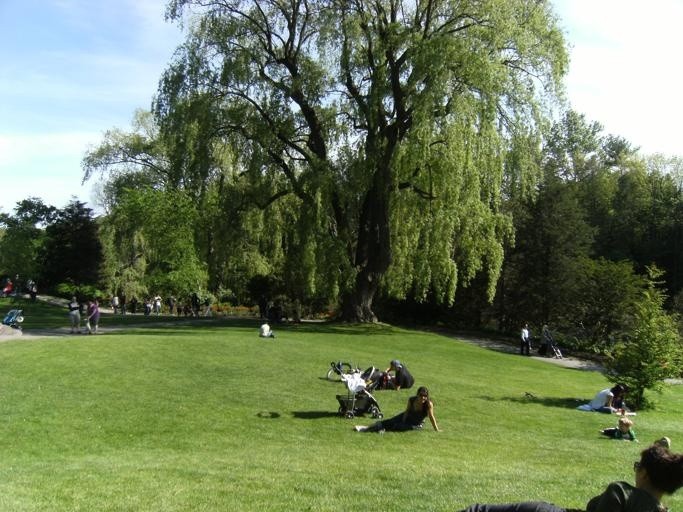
[520,324,532,356]
[538,325,553,357]
[388,359,414,390]
[592,383,632,415]
[258,318,272,338]
[354,386,443,434]
[455,446,683,512]
[0,275,213,336]
[603,417,641,443]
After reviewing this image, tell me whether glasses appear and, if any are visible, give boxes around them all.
[634,462,643,472]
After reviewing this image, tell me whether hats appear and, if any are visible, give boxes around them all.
[391,359,402,368]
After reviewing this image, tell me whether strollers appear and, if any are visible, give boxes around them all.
[548,339,562,359]
[330,362,383,420]
[2,300,24,331]
